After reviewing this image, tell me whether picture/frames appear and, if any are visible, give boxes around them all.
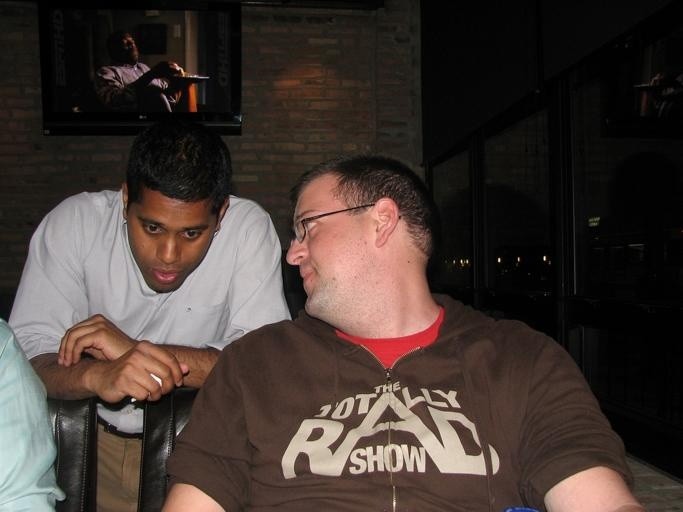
[37,1,242,138]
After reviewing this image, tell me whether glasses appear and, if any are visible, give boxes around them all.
[293,203,376,243]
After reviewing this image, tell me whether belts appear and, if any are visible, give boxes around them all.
[97,416,143,440]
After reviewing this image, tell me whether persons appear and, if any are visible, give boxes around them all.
[159,151,644,512]
[94,28,186,122]
[0,319,66,512]
[8,119,289,512]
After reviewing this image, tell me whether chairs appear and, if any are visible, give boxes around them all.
[38,395,100,511]
[133,380,204,511]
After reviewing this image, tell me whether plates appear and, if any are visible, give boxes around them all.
[167,75,210,84]
